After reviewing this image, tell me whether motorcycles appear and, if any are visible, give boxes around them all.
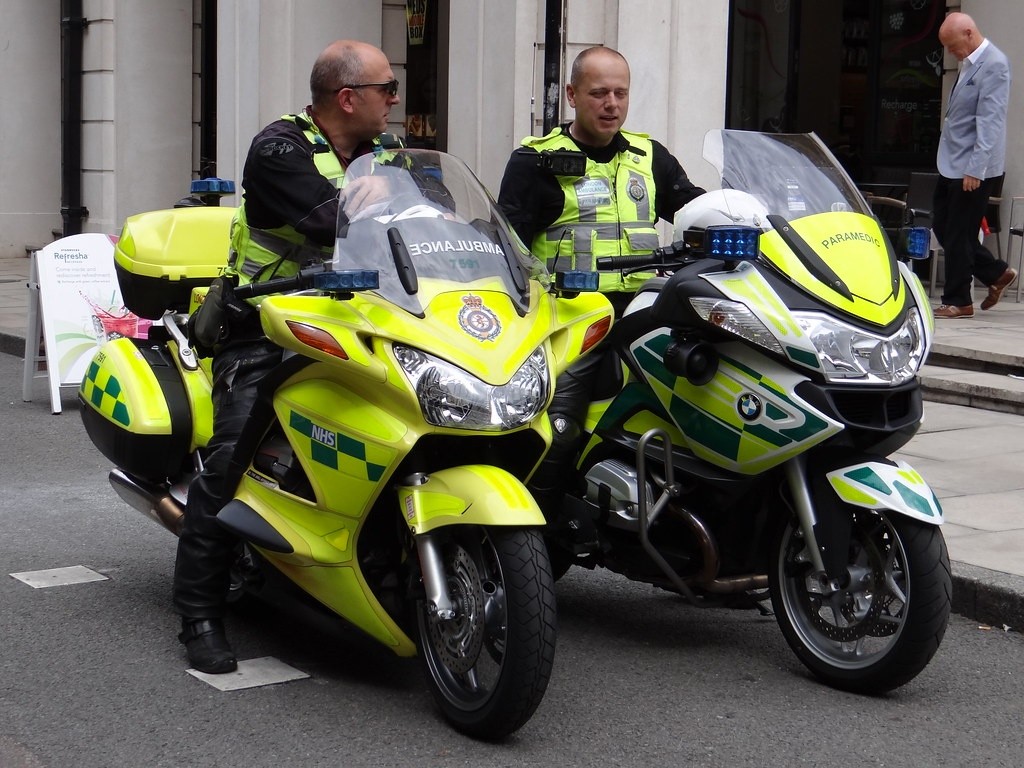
[73,146,604,743]
[533,129,954,697]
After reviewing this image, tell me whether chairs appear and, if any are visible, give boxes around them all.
[860,169,939,299]
[1002,196,1024,303]
[985,172,1007,257]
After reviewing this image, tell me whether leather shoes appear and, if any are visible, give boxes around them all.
[178,617,237,674]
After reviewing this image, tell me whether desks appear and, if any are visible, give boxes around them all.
[855,182,910,226]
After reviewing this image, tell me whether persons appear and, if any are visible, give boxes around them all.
[170,38,457,675]
[487,48,707,542]
[932,11,1018,318]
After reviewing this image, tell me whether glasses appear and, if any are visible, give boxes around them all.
[333,79,399,97]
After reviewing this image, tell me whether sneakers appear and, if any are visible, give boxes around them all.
[933,304,975,318]
[980,266,1017,310]
